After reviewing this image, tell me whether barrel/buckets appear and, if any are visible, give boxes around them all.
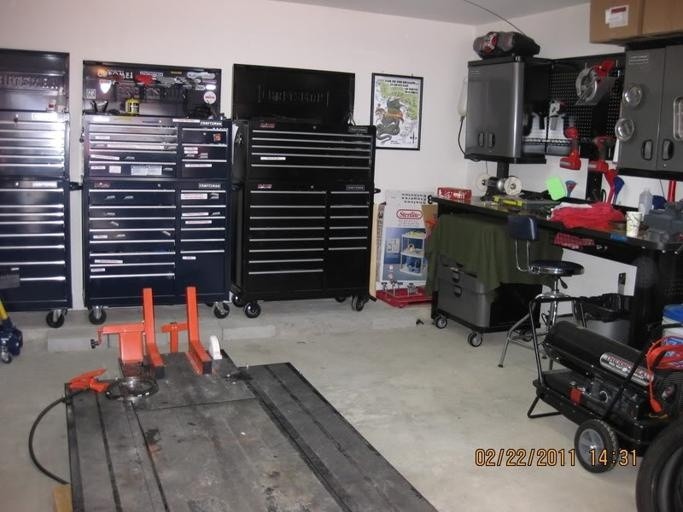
[661,304,682,364]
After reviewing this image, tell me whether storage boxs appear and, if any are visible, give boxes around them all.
[589,0,683,44]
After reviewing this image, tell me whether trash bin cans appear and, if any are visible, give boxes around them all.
[582,292,633,348]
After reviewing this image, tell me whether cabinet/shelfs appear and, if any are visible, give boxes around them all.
[611,45,683,181]
[464,61,550,163]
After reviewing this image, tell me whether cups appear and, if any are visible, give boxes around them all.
[625,211,643,238]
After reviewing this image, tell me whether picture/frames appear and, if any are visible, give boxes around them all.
[370,72,424,150]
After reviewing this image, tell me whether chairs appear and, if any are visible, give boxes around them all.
[497,214,589,385]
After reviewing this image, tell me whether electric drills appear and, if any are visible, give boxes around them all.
[583,135,615,174]
[559,128,582,170]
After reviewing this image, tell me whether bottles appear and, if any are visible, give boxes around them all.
[125,95,140,115]
[639,187,653,216]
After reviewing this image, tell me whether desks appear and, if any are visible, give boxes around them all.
[425,193,683,366]
